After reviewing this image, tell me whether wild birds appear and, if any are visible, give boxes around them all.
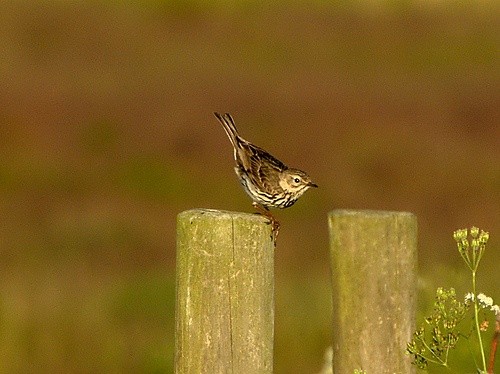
[212,112,319,248]
